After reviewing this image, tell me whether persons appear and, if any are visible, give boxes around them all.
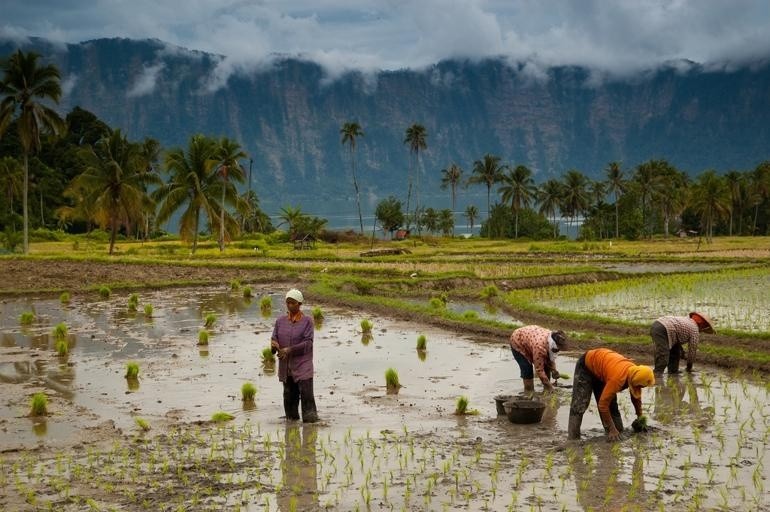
[511,326,565,391]
[652,310,716,380]
[270,291,317,422]
[568,350,653,442]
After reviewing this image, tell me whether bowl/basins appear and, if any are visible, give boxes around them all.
[502,400,546,425]
[494,395,528,416]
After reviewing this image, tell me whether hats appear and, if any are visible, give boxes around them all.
[689,311,718,336]
